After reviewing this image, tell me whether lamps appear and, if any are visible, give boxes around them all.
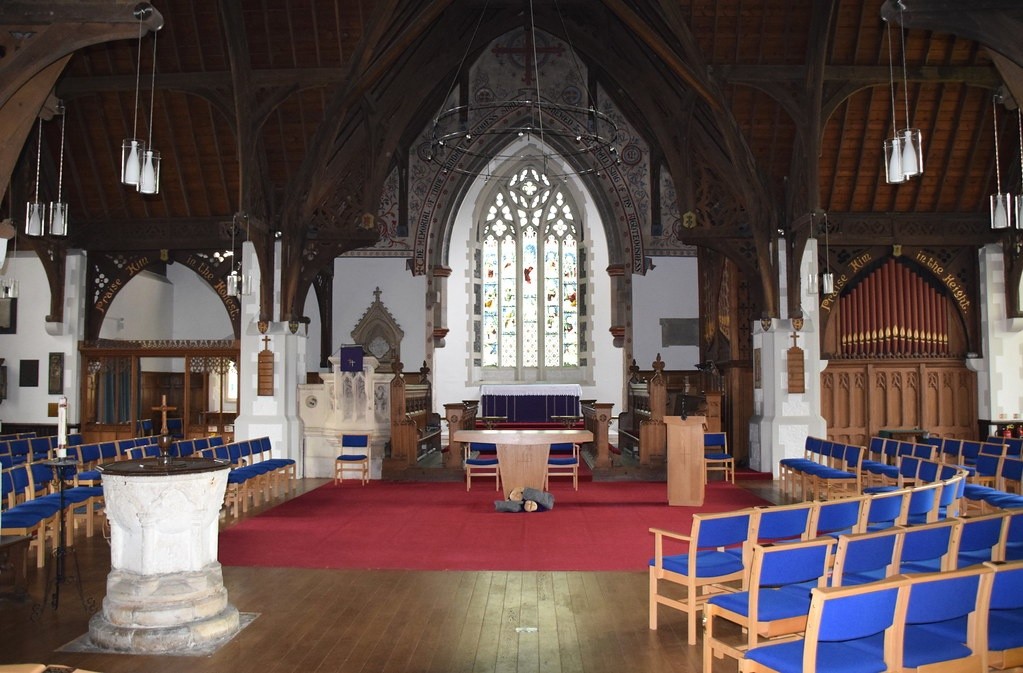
[25,105,69,236]
[0,218,20,299]
[227,212,237,295]
[807,213,834,294]
[990,93,1023,230]
[883,0,924,185]
[120,2,164,195]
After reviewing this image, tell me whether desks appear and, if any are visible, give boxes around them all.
[453,430,594,500]
[879,429,925,443]
[481,384,583,422]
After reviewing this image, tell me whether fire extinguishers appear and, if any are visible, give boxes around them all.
[1016,425,1023,437]
[1003,424,1014,438]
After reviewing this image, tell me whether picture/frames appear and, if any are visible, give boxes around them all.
[49,352,64,394]
[0,366,7,399]
[19,360,39,387]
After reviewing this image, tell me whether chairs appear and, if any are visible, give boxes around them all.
[0,414,297,568]
[334,433,371,485]
[544,442,580,491]
[649,429,1023,673]
[463,442,501,489]
